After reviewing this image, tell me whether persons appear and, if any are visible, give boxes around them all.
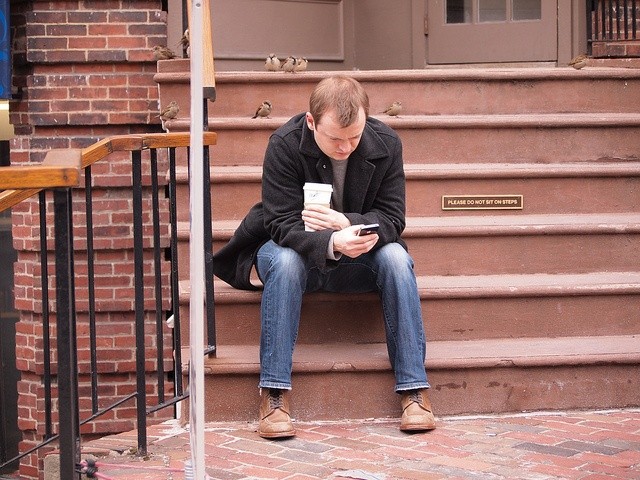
[213,75,436,439]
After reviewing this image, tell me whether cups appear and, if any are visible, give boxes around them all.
[303,183,333,233]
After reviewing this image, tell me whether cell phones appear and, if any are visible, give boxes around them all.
[357,223,380,236]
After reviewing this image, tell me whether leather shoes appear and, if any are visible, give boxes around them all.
[399,391,435,433]
[259,387,296,439]
